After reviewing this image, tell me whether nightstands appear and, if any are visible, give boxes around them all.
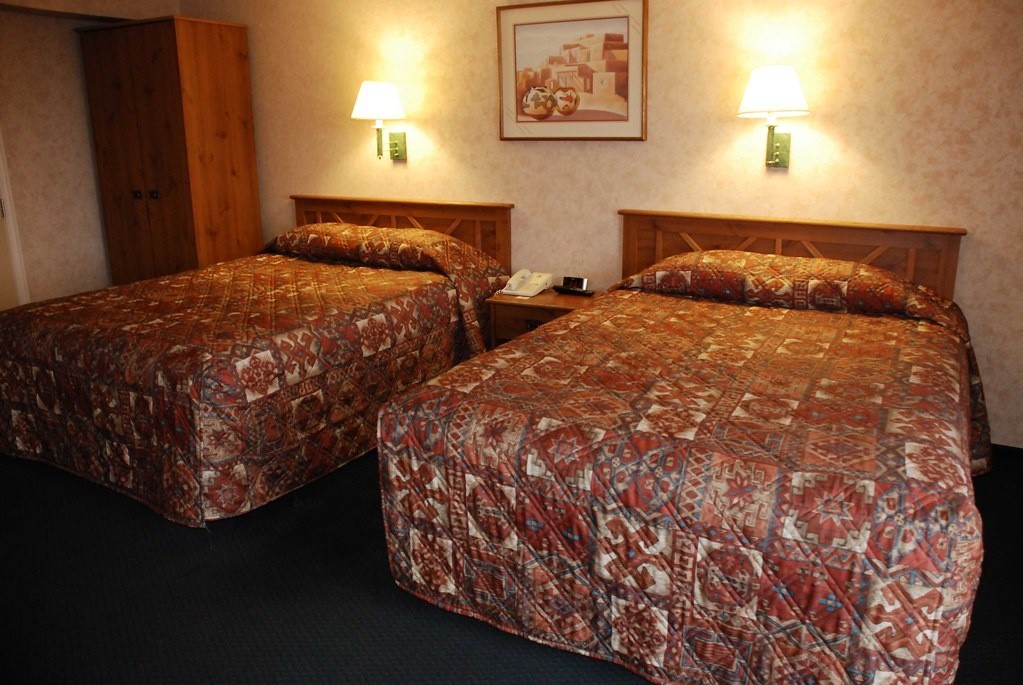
[484,287,605,349]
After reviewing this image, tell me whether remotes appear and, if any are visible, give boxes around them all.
[552,284,594,295]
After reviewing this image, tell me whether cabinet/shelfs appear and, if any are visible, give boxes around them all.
[74,13,265,288]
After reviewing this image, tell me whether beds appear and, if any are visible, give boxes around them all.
[0,195,514,531]
[375,209,994,685]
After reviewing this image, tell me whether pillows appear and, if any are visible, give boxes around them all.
[622,249,945,326]
[267,223,461,278]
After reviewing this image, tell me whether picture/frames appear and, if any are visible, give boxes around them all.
[495,0,649,143]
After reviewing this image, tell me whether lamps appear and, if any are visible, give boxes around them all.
[350,81,408,162]
[736,64,808,170]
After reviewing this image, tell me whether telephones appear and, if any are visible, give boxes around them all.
[495,269,553,297]
